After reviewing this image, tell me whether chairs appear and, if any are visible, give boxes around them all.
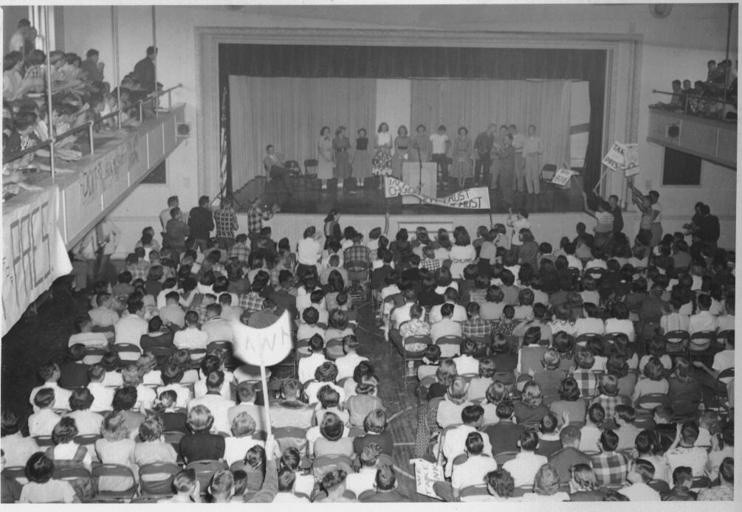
[539,164,557,194]
[265,159,319,190]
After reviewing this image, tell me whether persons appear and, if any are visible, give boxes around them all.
[1,18,738,502]
